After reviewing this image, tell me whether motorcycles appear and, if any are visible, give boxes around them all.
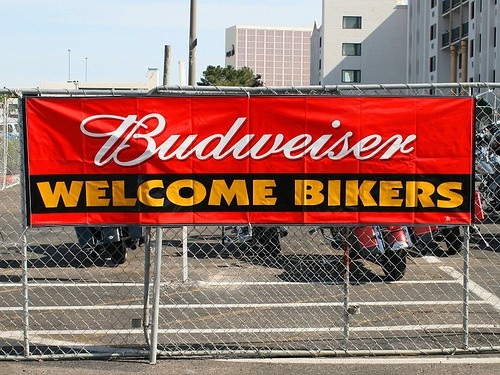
[475,130,500,223]
[307,225,412,279]
[76,225,146,263]
[405,194,484,254]
[223,224,289,255]
